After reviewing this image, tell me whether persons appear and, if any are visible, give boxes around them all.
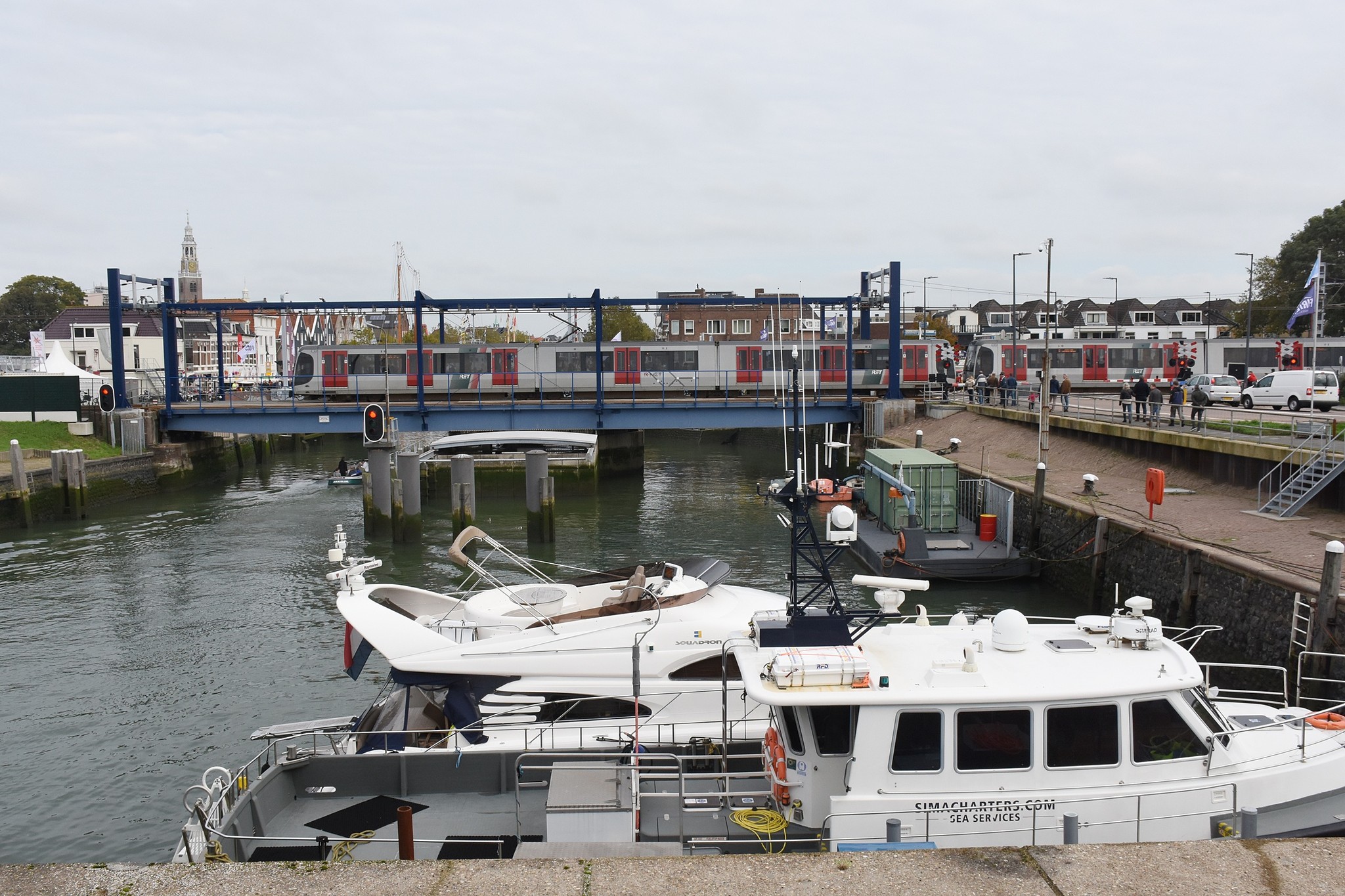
[1119,382,1137,424]
[1191,384,1208,432]
[1049,375,1061,412]
[1132,376,1151,421]
[1245,370,1257,387]
[1148,384,1164,427]
[1027,390,1039,410]
[964,371,1019,407]
[1168,381,1185,427]
[338,456,368,479]
[1058,373,1072,411]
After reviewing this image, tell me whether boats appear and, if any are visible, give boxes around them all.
[768,478,815,503]
[842,474,866,501]
[327,458,369,488]
[808,478,853,501]
[169,524,1345,867]
[324,280,1226,773]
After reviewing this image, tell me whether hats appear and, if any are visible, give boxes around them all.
[999,372,1005,375]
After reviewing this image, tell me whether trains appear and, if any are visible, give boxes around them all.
[963,338,1345,392]
[291,339,951,399]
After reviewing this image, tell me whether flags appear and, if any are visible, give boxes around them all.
[1302,255,1319,288]
[842,315,848,333]
[759,326,768,342]
[454,313,519,338]
[824,315,837,330]
[1286,281,1317,330]
[97,327,113,365]
[236,337,257,363]
[611,330,622,342]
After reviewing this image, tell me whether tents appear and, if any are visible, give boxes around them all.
[30,341,109,406]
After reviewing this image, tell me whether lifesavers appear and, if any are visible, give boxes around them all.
[771,744,786,802]
[762,727,777,778]
[1304,712,1345,731]
[897,531,906,555]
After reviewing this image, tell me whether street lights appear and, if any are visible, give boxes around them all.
[1103,277,1118,338]
[1054,299,1064,339]
[1234,252,1254,393]
[1079,318,1086,338]
[1013,252,1031,404]
[280,291,290,389]
[903,291,916,340]
[365,323,390,442]
[319,297,328,346]
[1043,291,1057,338]
[1204,291,1210,338]
[923,276,938,339]
[72,318,79,366]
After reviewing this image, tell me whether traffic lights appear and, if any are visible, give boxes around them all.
[1282,357,1298,366]
[1179,360,1185,365]
[99,383,115,412]
[945,362,949,367]
[364,404,384,442]
[1169,358,1176,366]
[1187,359,1195,367]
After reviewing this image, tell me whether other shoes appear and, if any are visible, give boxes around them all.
[967,401,1069,412]
[1122,418,1201,433]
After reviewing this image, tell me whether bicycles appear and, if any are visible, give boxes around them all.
[137,382,219,403]
[92,394,99,406]
[80,390,91,406]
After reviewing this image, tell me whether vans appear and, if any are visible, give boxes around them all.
[1240,370,1340,412]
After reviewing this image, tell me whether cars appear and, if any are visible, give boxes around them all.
[1181,374,1242,407]
